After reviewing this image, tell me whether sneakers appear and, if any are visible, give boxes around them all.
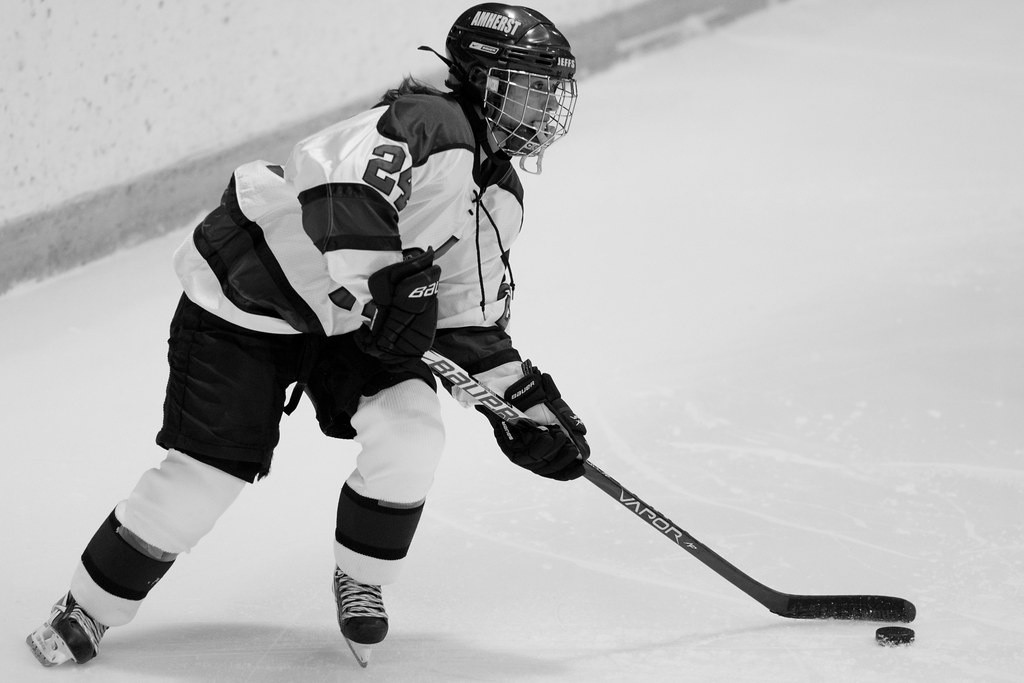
[27,592,108,668]
[333,565,388,667]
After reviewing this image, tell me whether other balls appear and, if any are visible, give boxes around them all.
[874,627,918,647]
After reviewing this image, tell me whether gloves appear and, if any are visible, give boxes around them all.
[353,247,441,364]
[473,349,590,481]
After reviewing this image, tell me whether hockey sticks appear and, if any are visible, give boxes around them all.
[420,348,921,626]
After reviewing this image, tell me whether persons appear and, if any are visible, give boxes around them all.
[24,0,592,668]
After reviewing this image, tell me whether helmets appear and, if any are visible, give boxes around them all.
[444,3,578,157]
